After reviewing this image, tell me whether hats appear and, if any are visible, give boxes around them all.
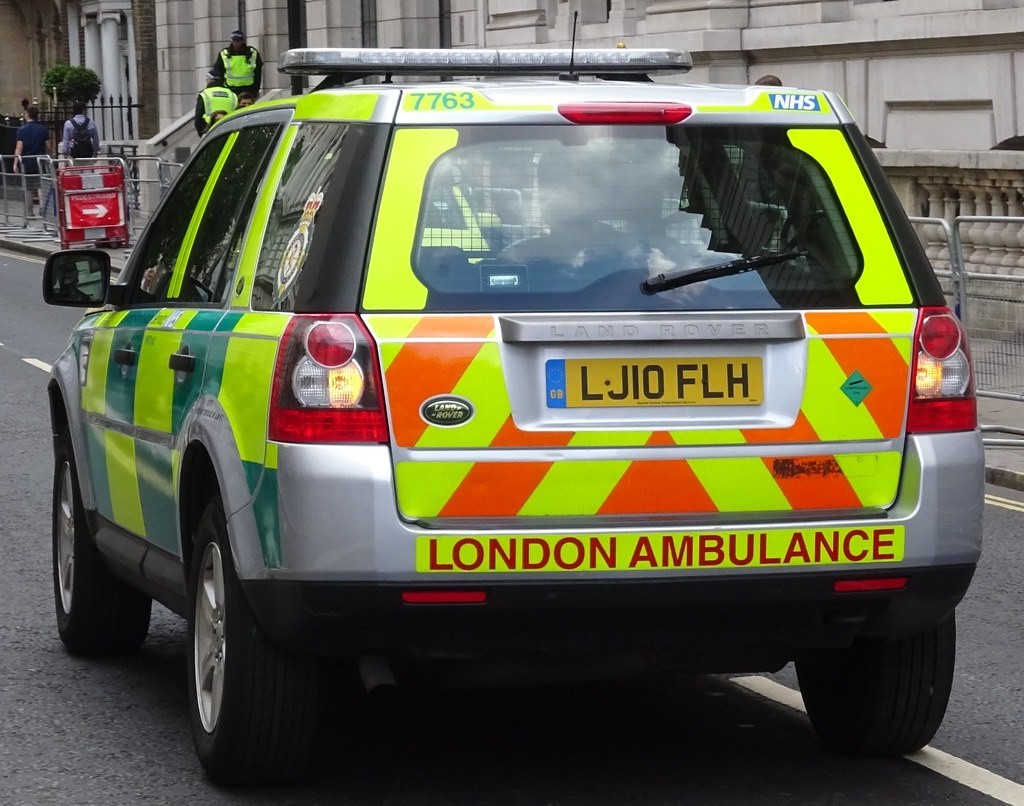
[231,30,245,39]
[208,70,222,80]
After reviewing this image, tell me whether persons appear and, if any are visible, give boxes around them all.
[208,30,263,99]
[63,102,101,173]
[194,70,238,140]
[13,105,51,220]
[238,91,255,107]
[210,110,228,128]
[496,150,630,267]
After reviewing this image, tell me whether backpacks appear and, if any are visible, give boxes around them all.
[70,120,94,159]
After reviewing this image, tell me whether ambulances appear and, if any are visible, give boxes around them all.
[42,46,985,789]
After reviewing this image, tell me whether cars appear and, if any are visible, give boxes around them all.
[420,185,503,264]
